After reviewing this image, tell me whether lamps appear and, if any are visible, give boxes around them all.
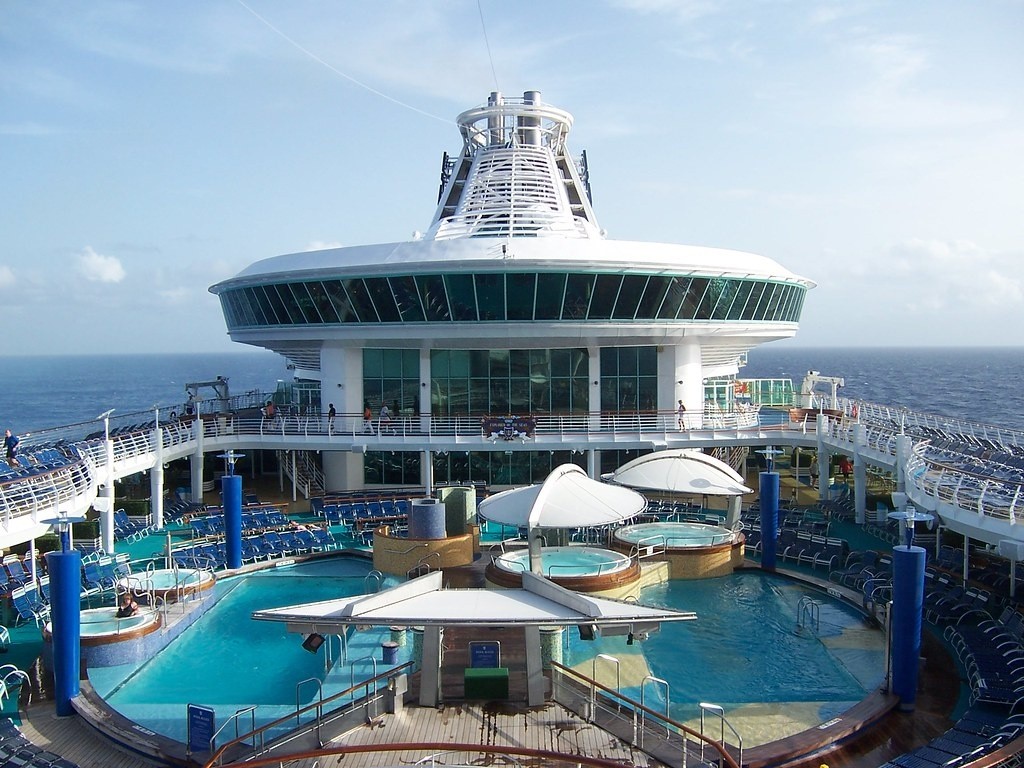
[577,625,600,641]
[302,623,326,655]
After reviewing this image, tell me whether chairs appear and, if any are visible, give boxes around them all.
[0,490,490,651]
[845,417,1024,519]
[0,717,82,768]
[0,417,176,510]
[518,485,1024,768]
[0,664,33,711]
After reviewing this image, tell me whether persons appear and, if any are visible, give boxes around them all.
[839,456,853,483]
[117,593,139,617]
[361,404,375,435]
[674,400,686,432]
[327,404,335,435]
[376,401,397,436]
[0,429,21,466]
[391,394,419,416]
[266,401,274,431]
[185,396,194,415]
[810,458,819,491]
